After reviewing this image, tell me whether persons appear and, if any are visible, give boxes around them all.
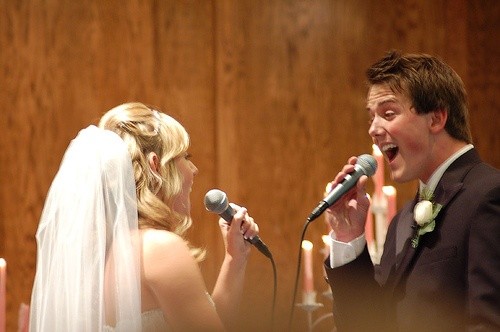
[76,102,259,332]
[323,52,500,332]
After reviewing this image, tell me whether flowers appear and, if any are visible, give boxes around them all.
[411,179,443,248]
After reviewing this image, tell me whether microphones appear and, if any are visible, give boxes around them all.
[306,154,378,222]
[203,188,273,259]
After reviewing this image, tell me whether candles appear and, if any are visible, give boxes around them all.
[372,143,385,200]
[301,240,318,303]
[364,198,375,256]
[0,257,7,332]
[323,245,330,277]
[18,302,29,332]
[383,185,398,223]
